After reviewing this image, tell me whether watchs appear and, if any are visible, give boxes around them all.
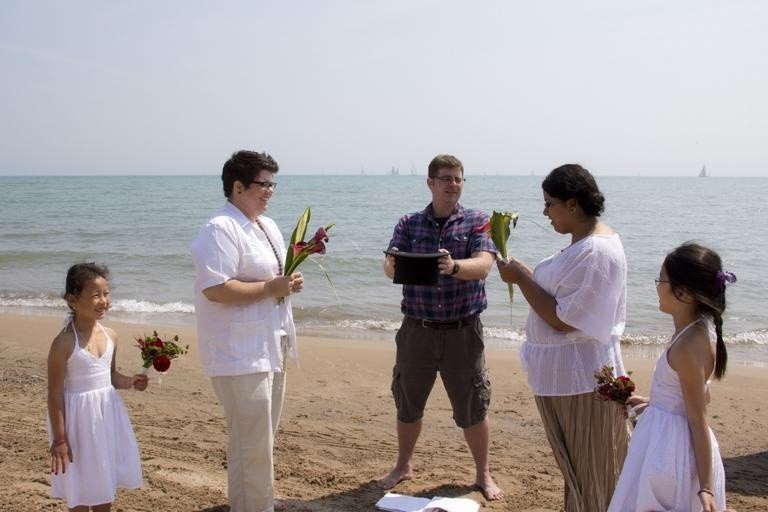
[449,260,459,276]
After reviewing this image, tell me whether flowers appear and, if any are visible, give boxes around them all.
[133,328,190,375]
[473,208,549,330]
[590,366,639,427]
[276,206,340,305]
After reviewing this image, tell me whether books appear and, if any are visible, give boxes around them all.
[376,491,480,512]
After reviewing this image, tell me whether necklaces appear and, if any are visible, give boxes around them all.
[74,323,100,352]
[255,219,282,276]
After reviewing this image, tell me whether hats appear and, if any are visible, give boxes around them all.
[383,250,453,286]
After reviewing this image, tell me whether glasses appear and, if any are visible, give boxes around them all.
[434,176,466,182]
[655,277,671,287]
[250,181,277,190]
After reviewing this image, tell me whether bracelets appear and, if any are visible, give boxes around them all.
[52,438,66,444]
[695,488,716,499]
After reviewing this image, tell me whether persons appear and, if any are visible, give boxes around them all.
[47,261,147,512]
[496,163,637,512]
[605,244,735,512]
[189,151,304,512]
[377,153,506,502]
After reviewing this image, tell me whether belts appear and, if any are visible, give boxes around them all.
[406,313,480,330]
